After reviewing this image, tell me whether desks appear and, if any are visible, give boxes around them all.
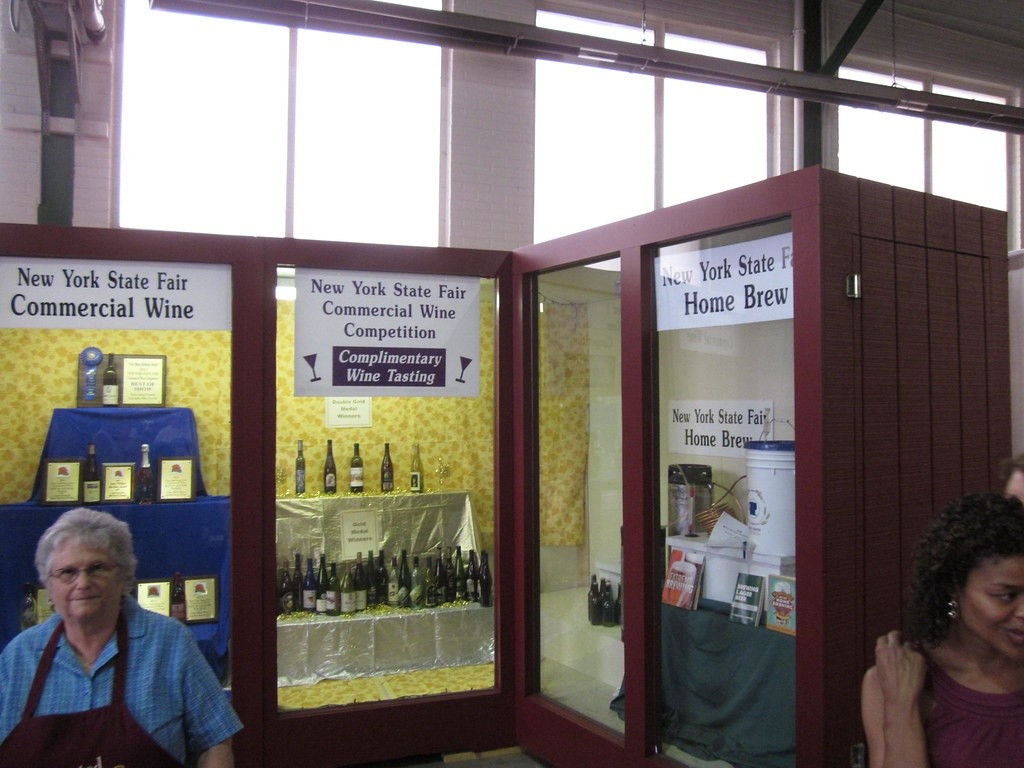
[658,533,796,768]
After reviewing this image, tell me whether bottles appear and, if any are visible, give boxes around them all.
[585,574,621,627]
[670,464,712,537]
[323,439,336,494]
[82,441,101,505]
[18,581,36,630]
[279,545,493,615]
[102,352,120,407]
[136,443,155,505]
[410,443,424,492]
[168,570,185,621]
[349,442,364,493]
[295,440,305,494]
[380,442,393,492]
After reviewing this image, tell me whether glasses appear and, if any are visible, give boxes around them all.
[48,561,122,581]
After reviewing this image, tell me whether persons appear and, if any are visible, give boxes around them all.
[996,451,1024,500]
[862,483,1024,768]
[0,507,243,768]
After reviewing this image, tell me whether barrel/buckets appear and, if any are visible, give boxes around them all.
[743,418,795,558]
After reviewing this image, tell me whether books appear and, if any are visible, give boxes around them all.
[767,574,796,637]
[728,573,766,627]
[660,550,707,611]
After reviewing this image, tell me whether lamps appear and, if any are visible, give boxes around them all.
[149,0,1024,137]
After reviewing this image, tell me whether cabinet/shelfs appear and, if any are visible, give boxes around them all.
[0,408,229,682]
[277,473,497,690]
